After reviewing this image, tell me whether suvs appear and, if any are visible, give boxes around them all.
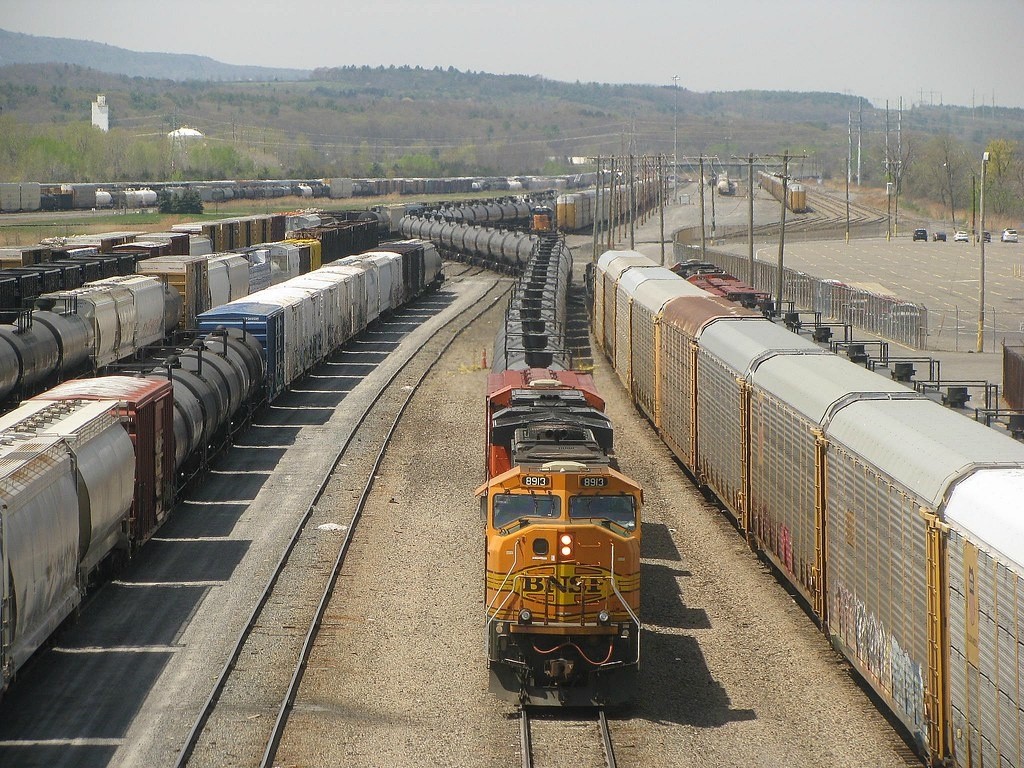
[913,229,927,241]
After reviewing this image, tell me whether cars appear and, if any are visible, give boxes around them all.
[1001,228,1018,242]
[977,231,991,242]
[933,232,946,242]
[954,231,969,242]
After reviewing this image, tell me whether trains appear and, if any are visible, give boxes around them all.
[589,244,1024,767]
[0,169,692,709]
[717,174,736,196]
[755,169,807,214]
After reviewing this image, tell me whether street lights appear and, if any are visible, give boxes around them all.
[978,151,992,351]
